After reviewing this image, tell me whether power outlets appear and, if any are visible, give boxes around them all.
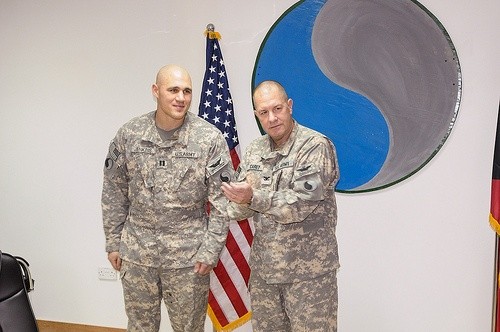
[97,268,117,280]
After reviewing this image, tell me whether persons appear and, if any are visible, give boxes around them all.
[99,63,235,332]
[218,79,341,332]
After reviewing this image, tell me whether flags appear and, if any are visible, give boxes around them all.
[195,24,255,332]
[489,100,500,332]
